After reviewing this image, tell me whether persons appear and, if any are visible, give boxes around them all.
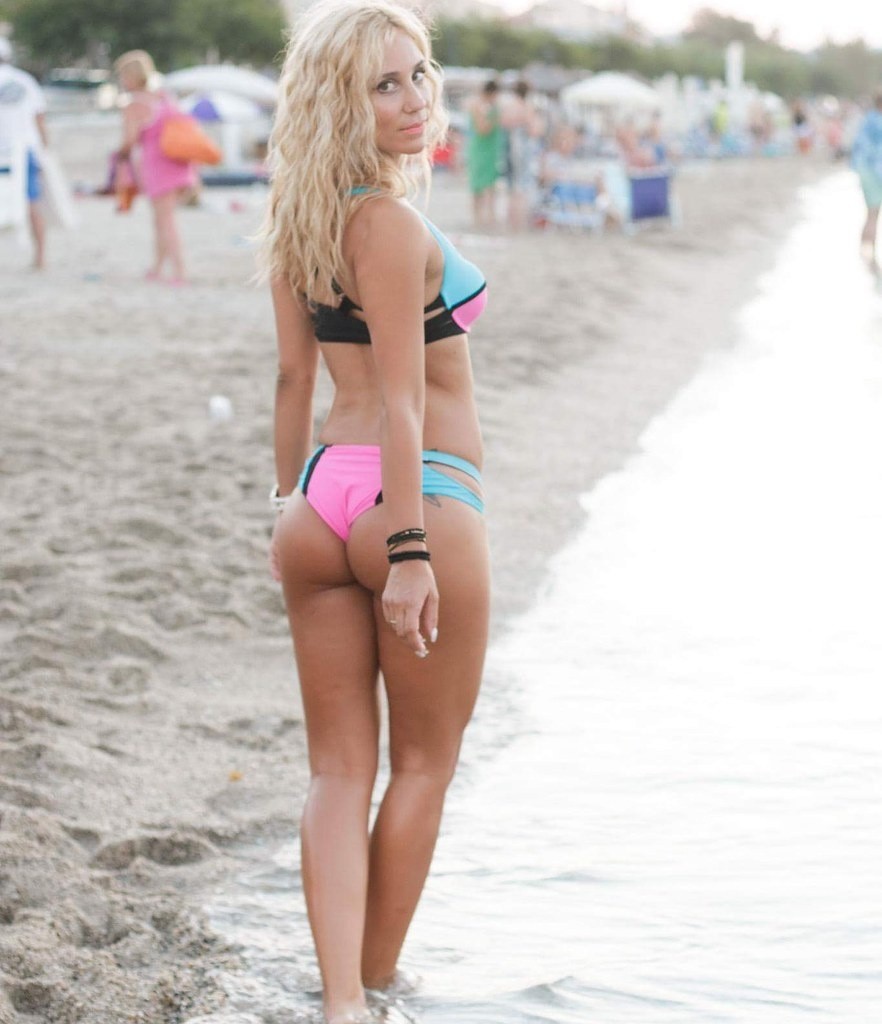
[115,51,201,285]
[268,0,491,1023]
[848,96,882,245]
[710,98,844,153]
[0,61,51,269]
[469,82,679,228]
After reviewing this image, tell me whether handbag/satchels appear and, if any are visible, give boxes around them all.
[161,113,220,163]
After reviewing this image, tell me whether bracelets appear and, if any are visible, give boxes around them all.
[387,550,431,565]
[386,528,427,552]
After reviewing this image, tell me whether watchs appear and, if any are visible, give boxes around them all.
[270,486,288,511]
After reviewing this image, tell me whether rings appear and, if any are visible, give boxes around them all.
[390,620,397,624]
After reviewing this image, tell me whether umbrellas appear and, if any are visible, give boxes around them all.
[160,62,279,123]
